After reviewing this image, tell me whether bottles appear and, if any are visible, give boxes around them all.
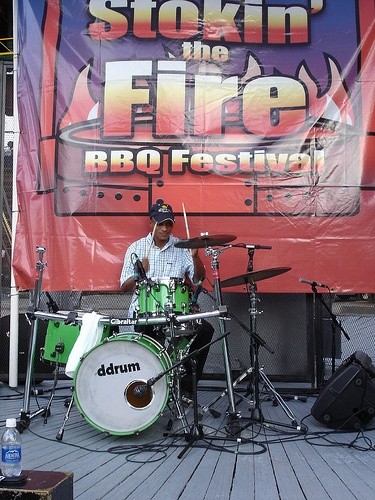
[0,418,23,478]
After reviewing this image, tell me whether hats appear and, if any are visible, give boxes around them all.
[150,203,174,224]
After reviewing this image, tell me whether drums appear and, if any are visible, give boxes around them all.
[136,276,190,318]
[41,309,116,367]
[156,337,190,367]
[72,331,175,436]
[160,303,201,336]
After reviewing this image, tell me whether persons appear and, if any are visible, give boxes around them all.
[119,203,215,408]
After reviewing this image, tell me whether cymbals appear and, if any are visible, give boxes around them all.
[212,266,292,288]
[173,234,237,249]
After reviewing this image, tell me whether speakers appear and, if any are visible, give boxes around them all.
[311,349,375,431]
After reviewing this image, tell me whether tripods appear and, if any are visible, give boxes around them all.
[146,245,307,458]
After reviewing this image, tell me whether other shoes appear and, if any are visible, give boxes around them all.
[180,391,193,408]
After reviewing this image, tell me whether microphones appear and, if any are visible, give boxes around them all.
[299,278,328,287]
[134,385,145,398]
[134,256,147,282]
